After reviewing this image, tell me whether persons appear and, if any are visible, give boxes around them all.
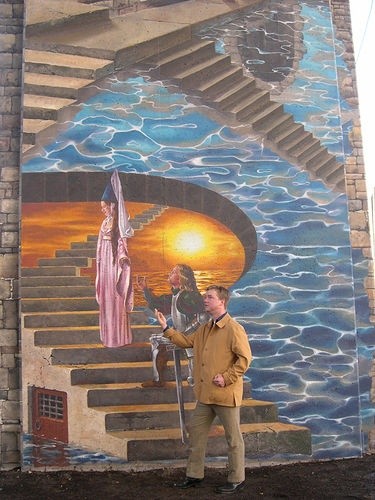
[155,285,252,494]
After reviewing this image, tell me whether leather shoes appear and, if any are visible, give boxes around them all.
[216,481,245,494]
[174,476,204,489]
